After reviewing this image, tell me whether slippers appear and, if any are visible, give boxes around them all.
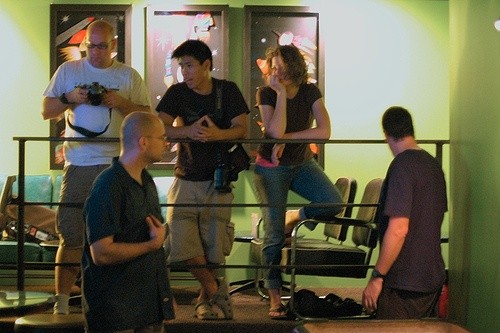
[284,210,291,246]
[268,305,287,319]
[212,280,233,319]
[193,301,218,319]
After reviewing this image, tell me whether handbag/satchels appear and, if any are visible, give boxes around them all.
[286,288,362,318]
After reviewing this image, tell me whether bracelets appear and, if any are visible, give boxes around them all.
[59,93,74,106]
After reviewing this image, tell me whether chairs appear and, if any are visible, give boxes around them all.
[0,175,178,307]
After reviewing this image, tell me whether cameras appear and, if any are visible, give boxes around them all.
[80,82,108,106]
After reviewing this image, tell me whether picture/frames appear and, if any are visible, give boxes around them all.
[50,4,131,169]
[243,4,325,171]
[146,4,229,170]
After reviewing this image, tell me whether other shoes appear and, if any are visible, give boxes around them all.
[53,293,69,315]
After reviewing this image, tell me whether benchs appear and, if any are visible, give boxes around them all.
[250,177,385,320]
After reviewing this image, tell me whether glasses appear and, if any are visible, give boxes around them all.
[85,40,112,50]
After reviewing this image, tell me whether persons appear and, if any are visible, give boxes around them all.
[155,39,250,321]
[361,107,448,321]
[42,20,155,315]
[251,44,345,321]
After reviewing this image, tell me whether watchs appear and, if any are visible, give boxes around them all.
[372,269,386,279]
[81,111,175,333]
